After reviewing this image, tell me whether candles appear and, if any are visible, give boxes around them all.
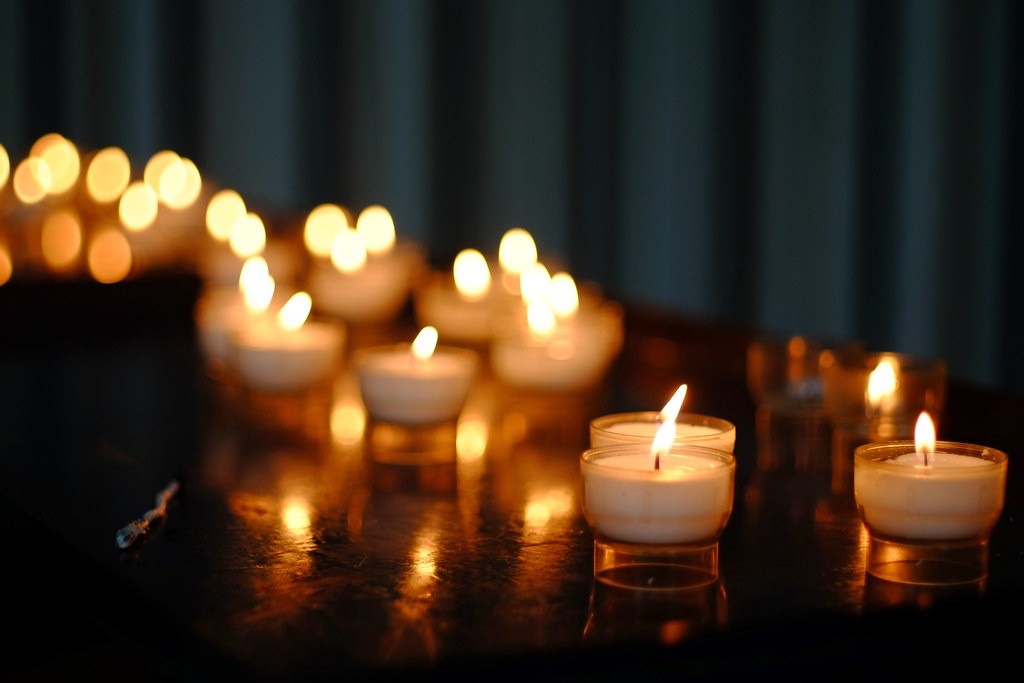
[853,440,1008,584]
[194,245,627,462]
[579,411,738,594]
